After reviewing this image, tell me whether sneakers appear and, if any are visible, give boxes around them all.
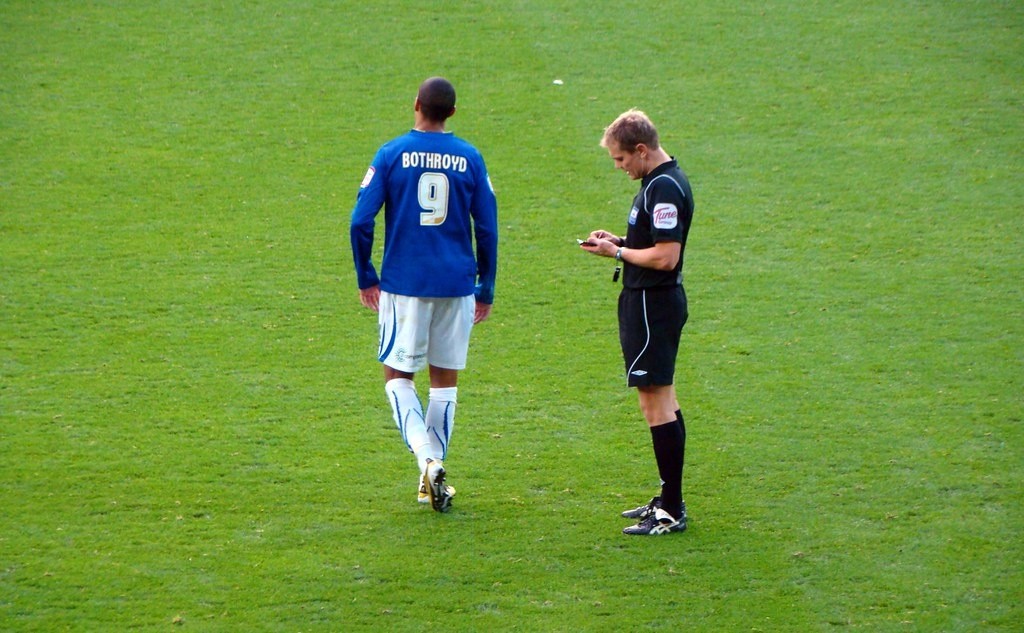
[418,473,456,502]
[426,459,452,513]
[621,496,686,534]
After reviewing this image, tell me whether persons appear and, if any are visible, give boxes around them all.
[349,76,499,513]
[579,107,696,536]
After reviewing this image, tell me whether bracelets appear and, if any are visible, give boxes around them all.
[616,246,627,262]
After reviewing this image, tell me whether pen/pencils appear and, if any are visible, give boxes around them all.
[597,232,603,239]
